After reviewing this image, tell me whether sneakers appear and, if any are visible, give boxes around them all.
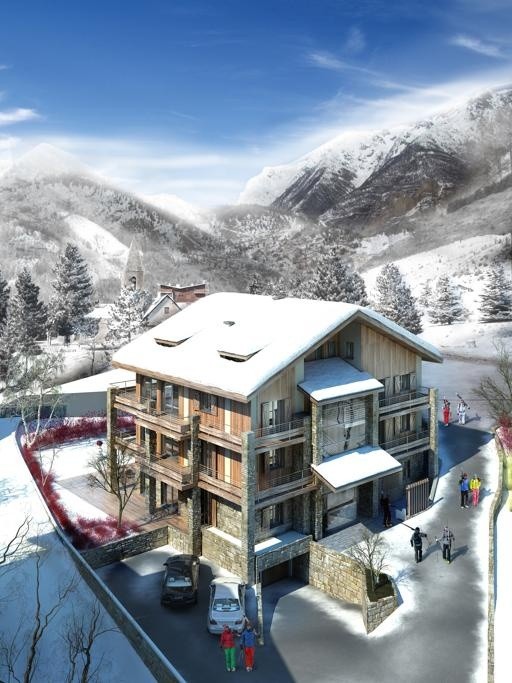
[460,505,470,510]
[225,666,255,673]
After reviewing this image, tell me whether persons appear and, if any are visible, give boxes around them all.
[457,472,471,509]
[441,401,452,428]
[456,400,471,425]
[433,527,457,565]
[470,473,481,507]
[381,494,395,529]
[239,622,260,674]
[218,624,238,674]
[410,526,427,564]
